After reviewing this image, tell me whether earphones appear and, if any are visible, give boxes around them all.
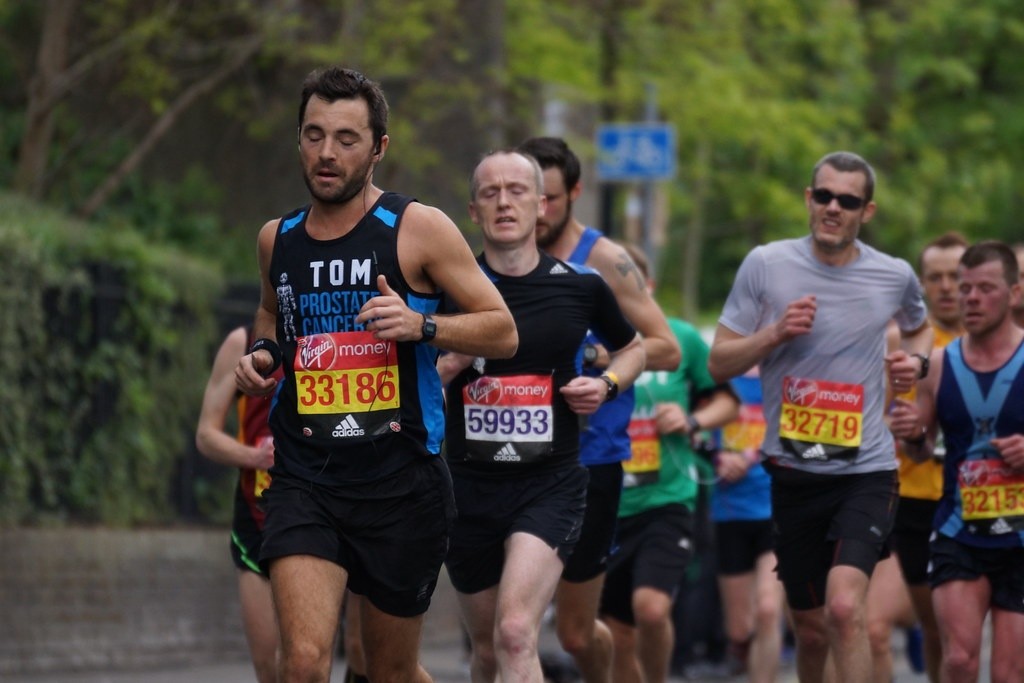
[374,146,381,156]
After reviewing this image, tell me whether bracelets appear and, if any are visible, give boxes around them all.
[248,338,281,374]
[603,370,620,386]
[903,427,928,445]
[686,414,701,430]
[695,440,719,461]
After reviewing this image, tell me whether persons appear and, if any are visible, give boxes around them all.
[433,149,648,683]
[192,312,289,683]
[704,150,936,682]
[513,137,683,683]
[230,63,519,682]
[595,232,1024,683]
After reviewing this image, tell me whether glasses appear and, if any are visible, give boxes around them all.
[812,188,862,209]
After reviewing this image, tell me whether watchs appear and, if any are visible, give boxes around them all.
[600,375,618,402]
[910,353,930,379]
[415,312,437,344]
